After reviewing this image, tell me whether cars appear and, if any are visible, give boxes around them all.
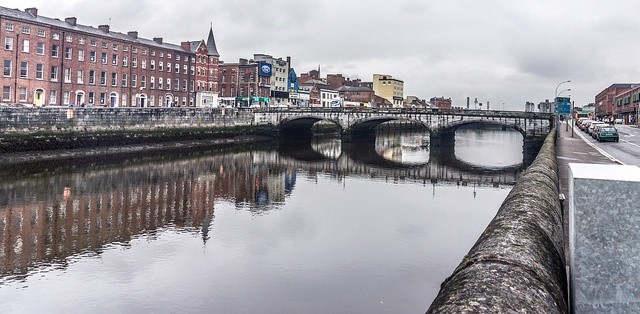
[597,125,619,142]
[585,121,591,133]
[588,122,605,136]
[577,117,588,129]
[580,119,593,131]
[592,124,609,139]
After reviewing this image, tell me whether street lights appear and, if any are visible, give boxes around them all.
[558,89,571,97]
[566,95,571,97]
[555,80,571,97]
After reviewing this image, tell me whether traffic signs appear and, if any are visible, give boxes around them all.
[556,97,570,115]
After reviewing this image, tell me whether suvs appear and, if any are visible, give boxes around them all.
[575,113,589,124]
